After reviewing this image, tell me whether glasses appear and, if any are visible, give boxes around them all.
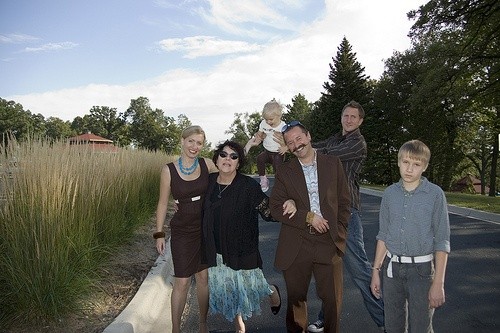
[219,150,238,160]
[281,120,305,133]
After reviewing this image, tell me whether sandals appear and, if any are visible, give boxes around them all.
[259,176,269,192]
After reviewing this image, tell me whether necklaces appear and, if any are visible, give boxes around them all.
[217,173,235,199]
[178,155,200,175]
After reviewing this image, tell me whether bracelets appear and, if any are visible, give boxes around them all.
[153,231,165,239]
[371,267,382,272]
[305,211,315,226]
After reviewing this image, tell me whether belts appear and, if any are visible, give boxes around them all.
[306,225,328,234]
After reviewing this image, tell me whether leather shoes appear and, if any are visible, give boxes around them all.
[270,284,282,315]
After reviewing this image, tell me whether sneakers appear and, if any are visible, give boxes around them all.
[307,319,325,332]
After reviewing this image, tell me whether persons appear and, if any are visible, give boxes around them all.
[172,140,297,333]
[269,120,352,333]
[370,140,451,333]
[155,124,262,333]
[244,97,289,193]
[254,99,385,333]
